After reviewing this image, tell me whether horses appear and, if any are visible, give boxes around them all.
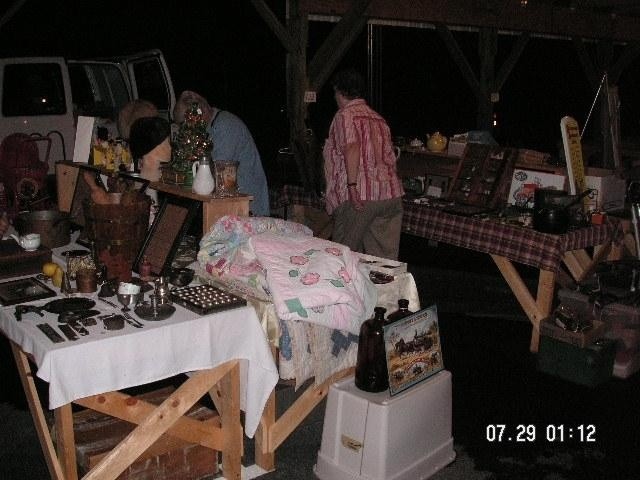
[395,342,414,359]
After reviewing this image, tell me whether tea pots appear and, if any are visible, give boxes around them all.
[424,131,448,153]
[9,233,41,252]
[192,154,214,196]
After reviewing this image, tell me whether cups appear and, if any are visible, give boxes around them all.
[76,268,98,293]
[214,158,240,198]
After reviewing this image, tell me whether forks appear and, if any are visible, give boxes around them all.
[124,311,145,326]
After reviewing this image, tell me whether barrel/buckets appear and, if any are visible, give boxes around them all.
[83,192,152,283]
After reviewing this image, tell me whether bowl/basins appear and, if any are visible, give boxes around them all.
[172,267,195,288]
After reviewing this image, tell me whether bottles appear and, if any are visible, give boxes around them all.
[137,255,152,278]
[155,275,171,304]
[356,298,413,394]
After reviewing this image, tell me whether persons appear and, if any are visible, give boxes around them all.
[322,68,405,262]
[1,211,11,241]
[129,116,173,182]
[173,91,272,217]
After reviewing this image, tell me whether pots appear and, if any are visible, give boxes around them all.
[17,209,73,249]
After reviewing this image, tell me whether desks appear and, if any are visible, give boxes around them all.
[1,183,640,480]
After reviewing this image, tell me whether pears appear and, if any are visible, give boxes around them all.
[43,263,63,287]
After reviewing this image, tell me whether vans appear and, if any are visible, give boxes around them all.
[0,48,176,200]
